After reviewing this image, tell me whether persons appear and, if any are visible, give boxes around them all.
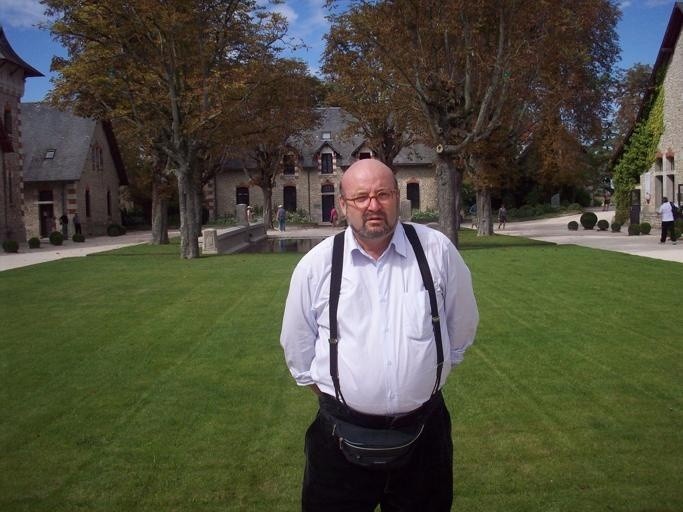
[601,188,611,211]
[247,206,255,222]
[469,202,478,229]
[276,205,286,232]
[41,211,82,240]
[497,204,507,230]
[278,159,480,512]
[655,197,677,245]
[331,206,338,227]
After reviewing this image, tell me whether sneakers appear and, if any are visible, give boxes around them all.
[658,240,677,245]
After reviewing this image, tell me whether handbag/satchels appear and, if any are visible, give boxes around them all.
[318,408,433,474]
[672,207,682,218]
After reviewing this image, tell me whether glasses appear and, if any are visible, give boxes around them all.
[345,190,395,209]
